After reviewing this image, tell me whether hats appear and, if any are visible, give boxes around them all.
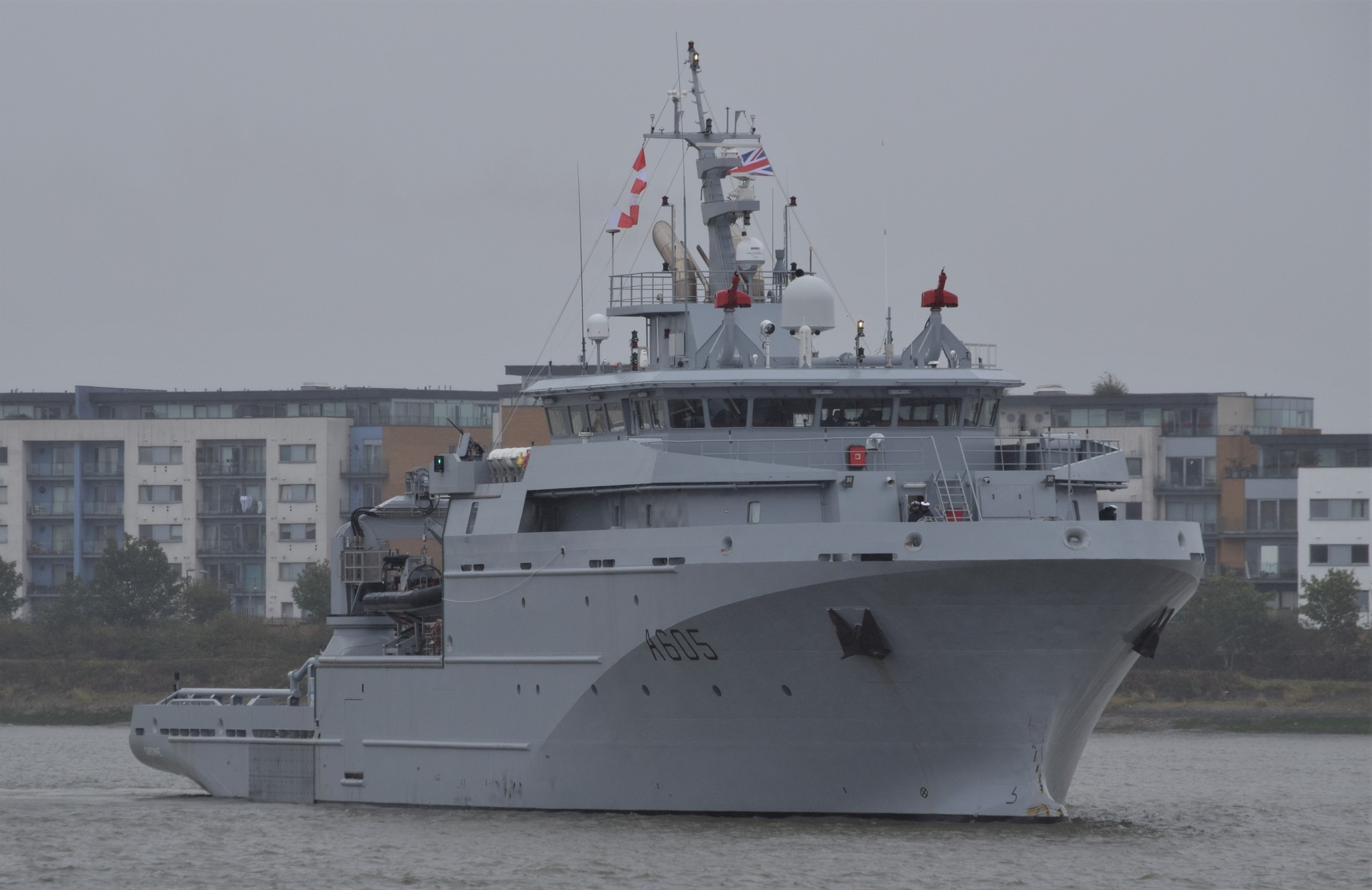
[1109,504,1117,510]
[921,500,929,507]
[911,501,920,506]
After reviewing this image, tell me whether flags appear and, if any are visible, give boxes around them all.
[727,147,774,176]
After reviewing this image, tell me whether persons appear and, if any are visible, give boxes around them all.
[908,501,934,522]
[824,407,903,426]
[1099,505,1118,520]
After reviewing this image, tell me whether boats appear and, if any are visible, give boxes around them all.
[130,32,1206,818]
